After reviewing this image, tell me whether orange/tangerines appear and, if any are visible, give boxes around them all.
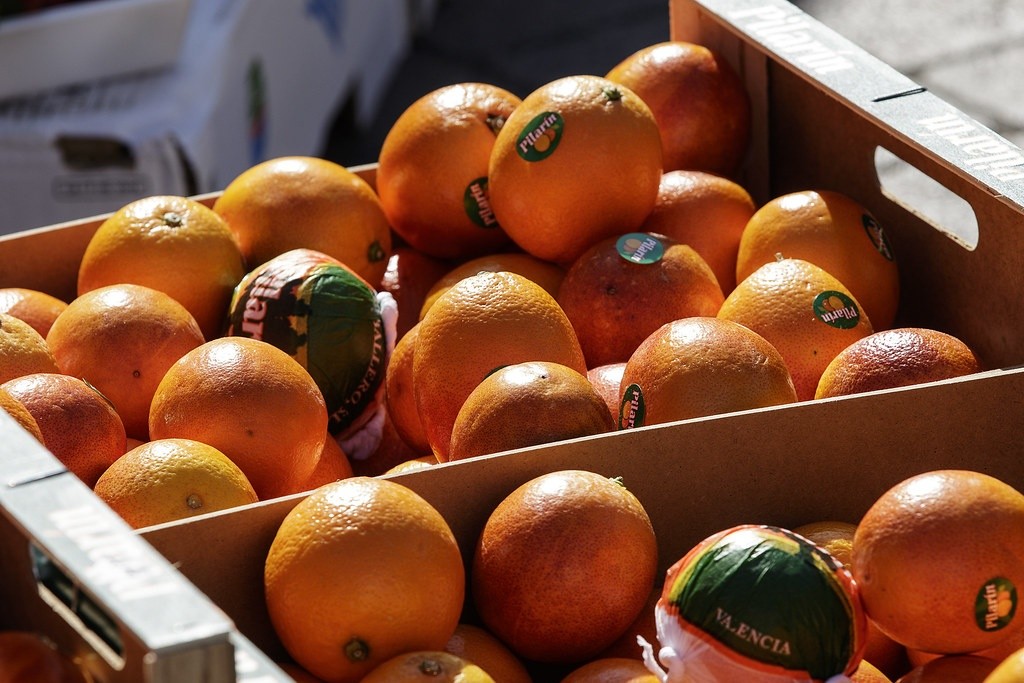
[0,37,1024,683]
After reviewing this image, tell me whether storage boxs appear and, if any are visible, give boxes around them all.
[1,1,1023,682]
